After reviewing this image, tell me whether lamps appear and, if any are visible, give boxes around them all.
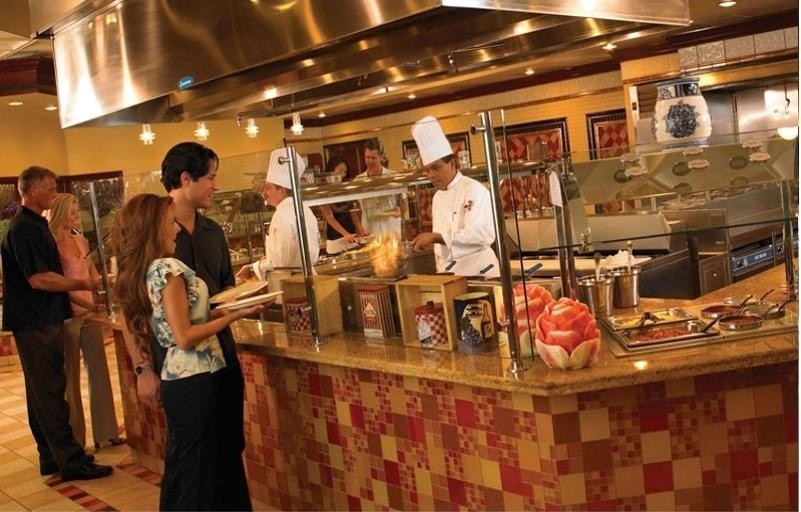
[139,121,159,149]
[194,120,212,143]
[244,117,262,140]
[290,113,305,138]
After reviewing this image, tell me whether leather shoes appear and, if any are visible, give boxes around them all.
[95,437,127,449]
[38,454,114,482]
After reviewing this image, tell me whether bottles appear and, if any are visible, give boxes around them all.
[649,77,713,153]
[287,295,312,335]
[414,300,448,345]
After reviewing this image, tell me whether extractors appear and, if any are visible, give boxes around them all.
[47,0,694,128]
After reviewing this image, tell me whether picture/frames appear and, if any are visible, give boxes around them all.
[401,128,474,222]
[584,106,636,214]
[494,116,574,222]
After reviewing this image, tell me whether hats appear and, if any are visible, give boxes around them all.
[265,146,307,192]
[411,115,456,168]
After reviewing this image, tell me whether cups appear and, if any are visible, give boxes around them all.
[454,290,496,346]
[576,265,641,319]
[458,149,470,170]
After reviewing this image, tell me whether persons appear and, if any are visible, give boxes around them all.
[407,153,501,279]
[116,193,275,512]
[1,166,115,479]
[40,191,128,453]
[250,178,320,305]
[318,154,368,254]
[350,138,406,242]
[118,142,253,512]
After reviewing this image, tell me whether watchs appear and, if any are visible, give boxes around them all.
[132,362,151,377]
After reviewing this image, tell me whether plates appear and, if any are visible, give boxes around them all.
[208,280,285,312]
